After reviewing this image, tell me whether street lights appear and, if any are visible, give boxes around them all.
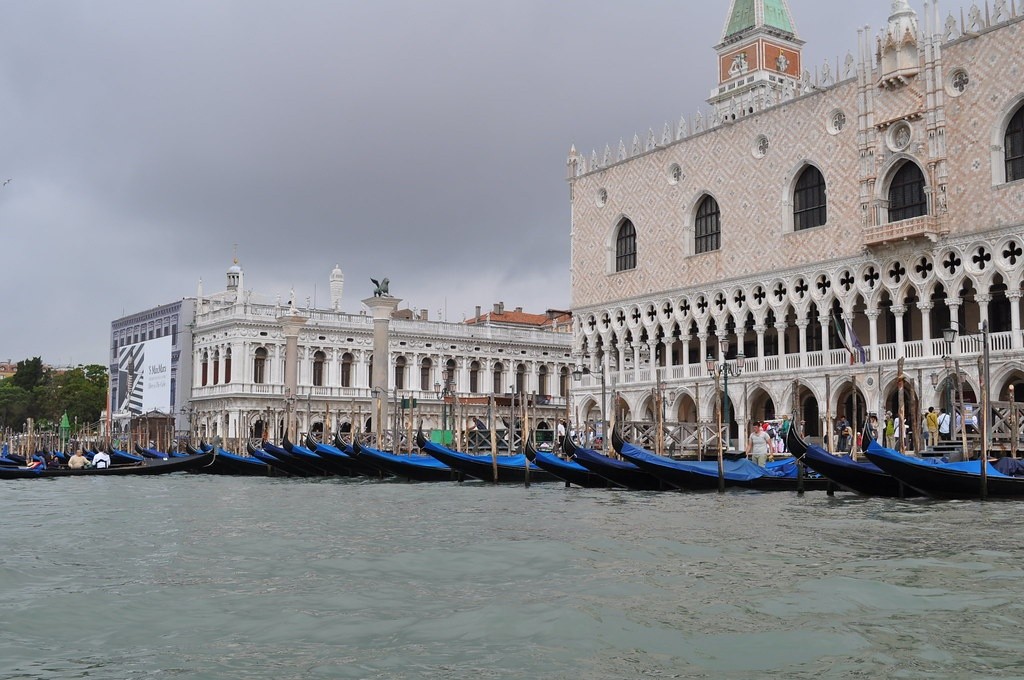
[706,338,746,444]
[280,387,295,435]
[180,400,193,446]
[941,318,990,447]
[371,384,398,455]
[434,369,456,446]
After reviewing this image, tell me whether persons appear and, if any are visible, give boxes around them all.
[92,446,111,468]
[937,408,950,440]
[68,450,91,469]
[779,415,789,453]
[468,417,486,431]
[571,429,575,441]
[857,432,862,452]
[862,412,879,450]
[558,419,565,449]
[835,415,849,452]
[746,421,773,467]
[758,421,781,452]
[49,457,64,470]
[42,447,51,463]
[540,441,549,451]
[922,412,929,450]
[927,406,938,445]
[51,450,65,464]
[584,430,586,442]
[883,410,909,451]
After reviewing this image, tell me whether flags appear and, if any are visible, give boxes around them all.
[844,317,865,364]
[833,315,855,365]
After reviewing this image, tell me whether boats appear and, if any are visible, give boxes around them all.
[0,413,1024,495]
